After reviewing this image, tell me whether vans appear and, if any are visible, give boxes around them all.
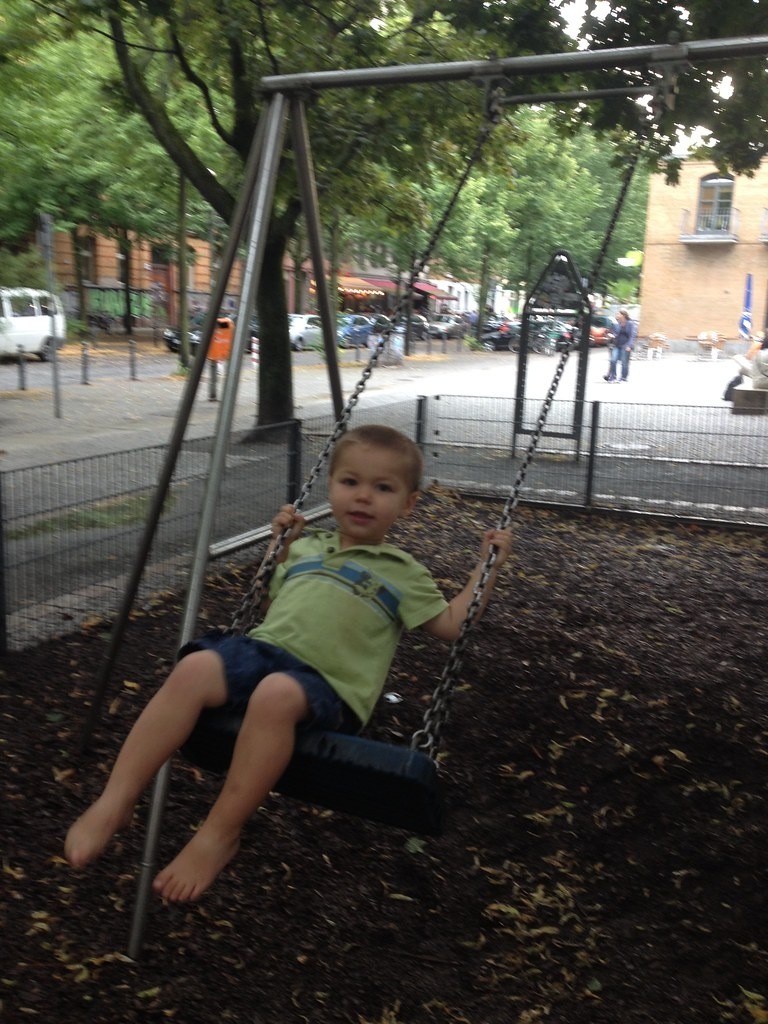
[0,287,66,361]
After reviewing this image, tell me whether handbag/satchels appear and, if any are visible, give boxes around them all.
[752,348,768,389]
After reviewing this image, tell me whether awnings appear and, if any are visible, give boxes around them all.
[410,281,459,300]
[329,274,385,297]
[364,277,424,300]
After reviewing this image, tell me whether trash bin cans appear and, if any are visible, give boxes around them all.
[206,318,235,362]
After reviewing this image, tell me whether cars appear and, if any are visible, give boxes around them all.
[468,309,622,352]
[362,313,430,342]
[333,313,374,349]
[162,310,259,356]
[426,314,471,340]
[288,313,323,352]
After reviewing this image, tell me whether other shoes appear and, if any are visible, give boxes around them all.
[617,377,627,383]
[604,376,616,381]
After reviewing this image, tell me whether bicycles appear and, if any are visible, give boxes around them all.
[507,328,550,354]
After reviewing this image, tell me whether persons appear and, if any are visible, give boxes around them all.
[743,331,768,388]
[61,418,513,903]
[604,311,636,380]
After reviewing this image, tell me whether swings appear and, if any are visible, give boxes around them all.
[174,112,653,837]
